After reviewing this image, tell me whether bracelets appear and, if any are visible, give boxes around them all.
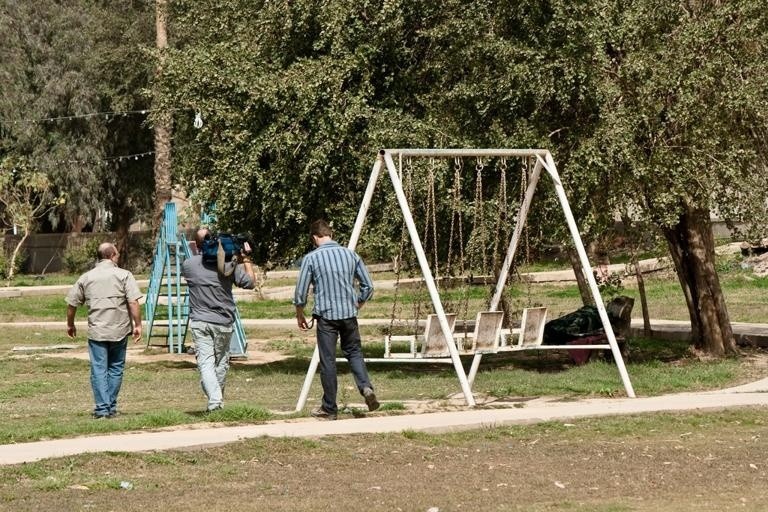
[242,260,252,265]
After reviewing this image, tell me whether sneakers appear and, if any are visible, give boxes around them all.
[362,385,382,412]
[309,405,339,421]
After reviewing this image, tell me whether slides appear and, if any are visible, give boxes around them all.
[180,235,249,356]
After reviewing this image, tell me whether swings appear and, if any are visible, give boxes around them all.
[384,157,548,359]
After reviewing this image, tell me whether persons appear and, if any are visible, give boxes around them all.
[63,242,144,420]
[292,218,381,421]
[180,227,257,414]
